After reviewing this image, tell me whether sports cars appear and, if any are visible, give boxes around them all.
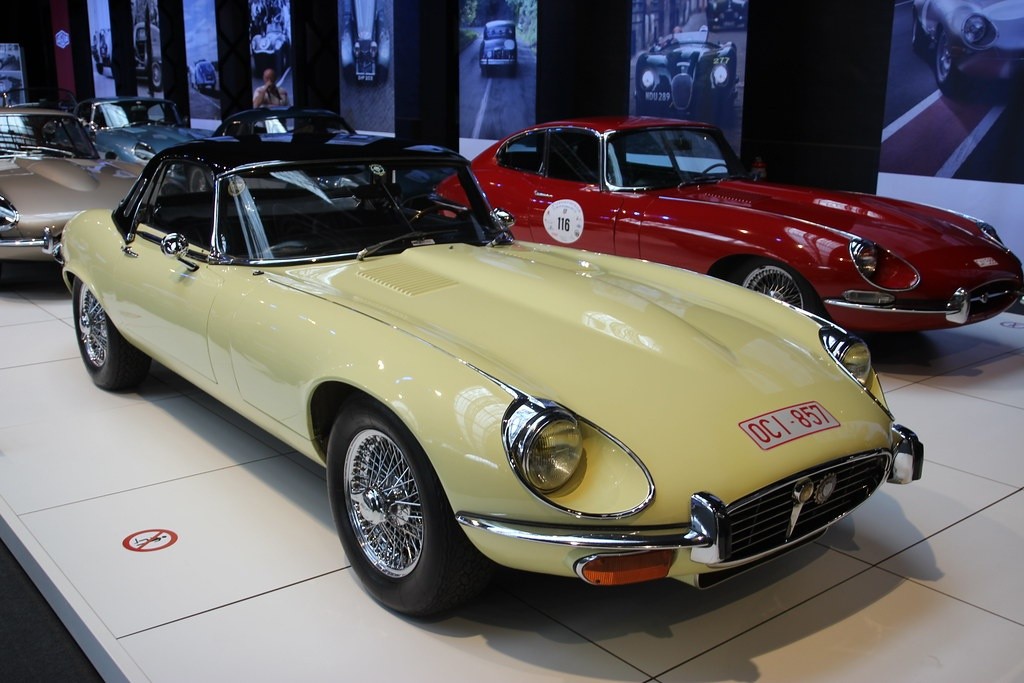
[434,119,1024,339]
[58,141,925,619]
[0,107,185,271]
[45,96,225,166]
[184,107,470,201]
[636,31,737,117]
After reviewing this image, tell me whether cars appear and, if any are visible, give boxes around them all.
[912,0,1023,95]
[479,21,519,73]
[250,21,292,70]
[92,23,164,92]
[354,35,378,81]
[190,58,218,92]
[0,53,15,68]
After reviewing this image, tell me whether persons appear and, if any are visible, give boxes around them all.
[253,68,288,132]
